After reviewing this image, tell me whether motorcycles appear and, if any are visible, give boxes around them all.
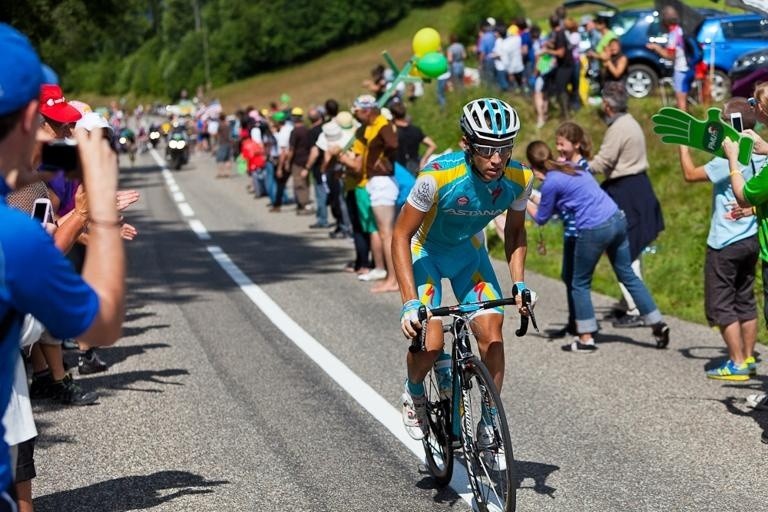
[164,117,189,171]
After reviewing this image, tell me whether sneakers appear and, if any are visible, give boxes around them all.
[400,378,430,440]
[50,374,98,404]
[63,338,79,349]
[476,423,508,470]
[605,308,625,322]
[760,430,768,443]
[747,359,756,375]
[612,314,646,328]
[78,352,108,375]
[747,393,768,407]
[652,322,670,349]
[29,369,54,399]
[561,341,596,353]
[706,361,750,380]
[361,271,388,281]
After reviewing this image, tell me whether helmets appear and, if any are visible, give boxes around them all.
[281,94,289,103]
[271,111,286,122]
[664,5,680,26]
[291,107,305,116]
[460,98,520,144]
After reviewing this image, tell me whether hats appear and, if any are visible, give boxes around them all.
[333,111,362,135]
[0,21,43,113]
[350,95,380,115]
[317,121,351,156]
[68,100,93,121]
[40,64,60,85]
[249,109,261,121]
[38,84,82,123]
[77,113,114,139]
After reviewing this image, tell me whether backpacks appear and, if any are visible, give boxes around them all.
[682,31,704,68]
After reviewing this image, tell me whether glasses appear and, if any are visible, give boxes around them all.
[470,143,514,158]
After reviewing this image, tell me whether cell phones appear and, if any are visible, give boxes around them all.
[730,112,743,135]
[29,196,52,231]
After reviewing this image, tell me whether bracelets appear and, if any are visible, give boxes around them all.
[87,214,127,232]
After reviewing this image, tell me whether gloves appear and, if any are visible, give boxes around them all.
[399,300,429,325]
[512,282,539,311]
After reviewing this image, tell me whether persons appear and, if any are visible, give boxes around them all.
[361,7,705,128]
[41,85,108,374]
[391,98,539,472]
[721,128,768,444]
[27,114,100,404]
[4,313,44,511]
[551,122,593,341]
[526,140,670,353]
[674,98,768,381]
[1,185,87,512]
[0,21,139,512]
[132,66,437,294]
[555,82,665,328]
[752,82,768,126]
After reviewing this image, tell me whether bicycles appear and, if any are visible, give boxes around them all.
[409,289,540,512]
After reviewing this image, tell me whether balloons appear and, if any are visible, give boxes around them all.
[412,27,440,56]
[416,52,450,79]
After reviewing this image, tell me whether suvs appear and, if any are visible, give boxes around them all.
[563,0,722,103]
[692,12,768,103]
